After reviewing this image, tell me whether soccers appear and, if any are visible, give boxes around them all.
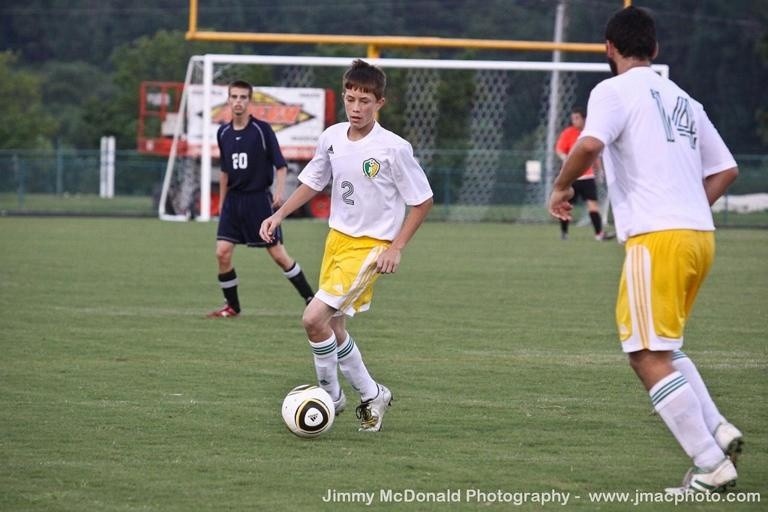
[281,384,335,437]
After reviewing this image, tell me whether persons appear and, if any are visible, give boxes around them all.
[555,106,617,242]
[546,4,744,500]
[258,60,435,435]
[203,79,318,320]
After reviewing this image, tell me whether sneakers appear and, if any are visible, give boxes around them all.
[354,383,394,434]
[331,387,347,416]
[205,304,241,319]
[661,420,744,501]
